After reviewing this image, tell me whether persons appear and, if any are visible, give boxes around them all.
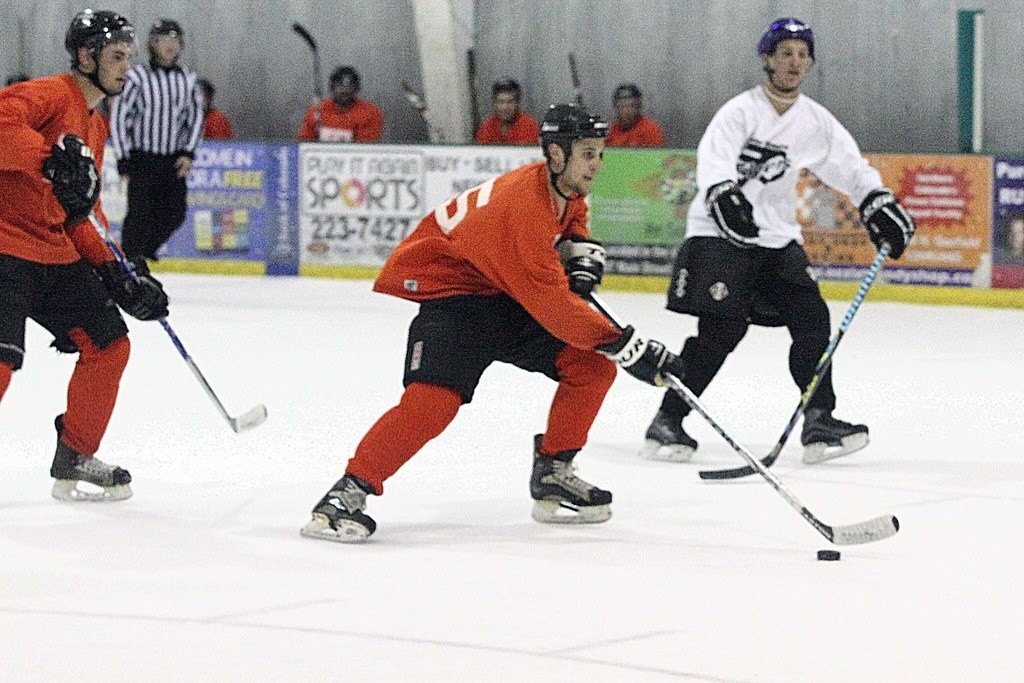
[604,83,665,149]
[298,104,686,544]
[109,17,205,273]
[645,17,918,464]
[475,77,540,146]
[299,66,382,143]
[194,79,232,139]
[0,8,170,502]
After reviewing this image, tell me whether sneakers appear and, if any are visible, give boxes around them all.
[50,414,133,501]
[300,473,377,543]
[801,405,870,464]
[530,433,612,524]
[644,408,698,463]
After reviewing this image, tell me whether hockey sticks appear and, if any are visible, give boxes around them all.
[88,209,268,433]
[404,83,447,143]
[699,239,892,480]
[587,292,900,546]
[568,52,582,104]
[467,49,483,136]
[292,20,323,140]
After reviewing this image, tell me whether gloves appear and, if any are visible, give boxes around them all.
[555,235,606,301]
[858,187,915,260]
[98,260,169,321]
[41,134,101,227]
[705,180,759,249]
[594,324,685,387]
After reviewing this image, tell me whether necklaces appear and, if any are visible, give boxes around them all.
[764,86,796,103]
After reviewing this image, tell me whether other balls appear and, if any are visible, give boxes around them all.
[817,549,841,561]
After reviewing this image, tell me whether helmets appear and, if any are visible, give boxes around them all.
[64,9,136,51]
[151,19,182,37]
[538,104,607,141]
[329,67,359,87]
[492,80,521,97]
[614,85,642,98]
[759,18,814,57]
